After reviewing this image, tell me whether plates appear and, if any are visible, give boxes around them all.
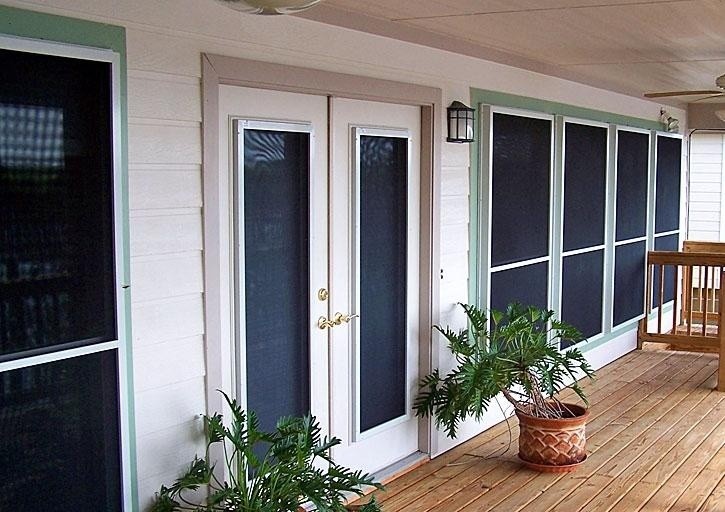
[516,452,588,472]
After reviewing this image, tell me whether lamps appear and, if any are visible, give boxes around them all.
[444,101,477,146]
[668,125,679,133]
[660,108,678,128]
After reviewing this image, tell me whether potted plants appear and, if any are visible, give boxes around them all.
[412,297,599,474]
